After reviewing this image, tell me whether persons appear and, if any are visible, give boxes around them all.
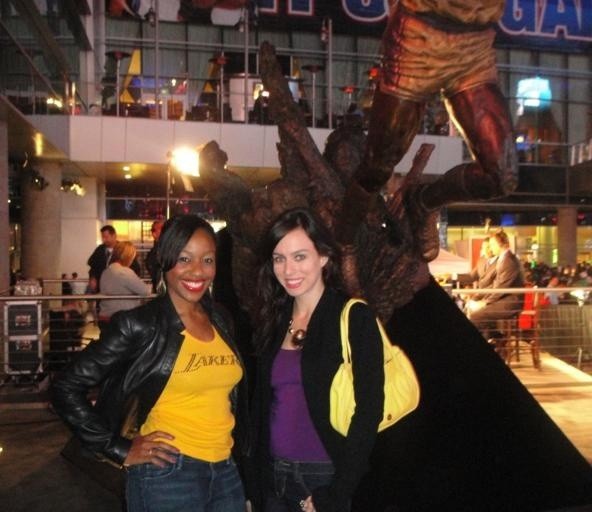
[61,273,75,305]
[334,1,520,304]
[462,230,526,341]
[69,272,86,296]
[96,240,152,337]
[526,260,592,289]
[144,220,167,294]
[243,204,386,509]
[47,212,256,511]
[87,225,141,313]
[254,49,441,326]
[467,237,499,289]
[10,269,28,295]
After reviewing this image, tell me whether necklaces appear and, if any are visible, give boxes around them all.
[288,319,309,350]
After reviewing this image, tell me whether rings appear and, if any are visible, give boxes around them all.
[148,449,154,456]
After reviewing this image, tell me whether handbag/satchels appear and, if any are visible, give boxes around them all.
[327,298,423,439]
